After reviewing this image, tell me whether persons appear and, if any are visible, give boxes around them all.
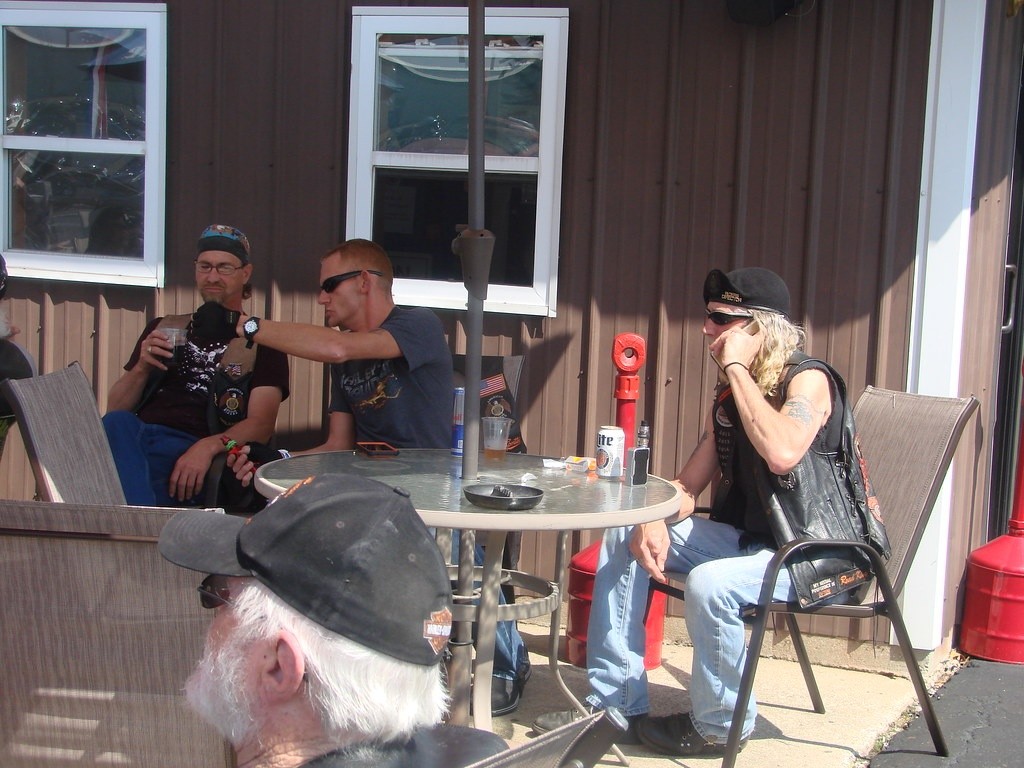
[530,266,893,758]
[156,472,530,768]
[100,223,290,519]
[0,252,21,343]
[185,238,533,719]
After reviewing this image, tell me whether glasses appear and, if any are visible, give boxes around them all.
[197,573,311,685]
[704,308,753,324]
[320,270,384,294]
[193,259,243,275]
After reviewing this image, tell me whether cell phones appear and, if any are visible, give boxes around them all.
[356,441,400,456]
[711,320,760,371]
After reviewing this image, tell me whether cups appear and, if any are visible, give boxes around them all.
[159,328,188,370]
[481,417,513,463]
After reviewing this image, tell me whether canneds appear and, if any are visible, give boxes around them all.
[450,387,465,457]
[596,425,624,478]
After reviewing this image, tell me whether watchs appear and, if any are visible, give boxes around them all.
[242,316,261,349]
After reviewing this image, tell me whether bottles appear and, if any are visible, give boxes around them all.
[637,421,651,447]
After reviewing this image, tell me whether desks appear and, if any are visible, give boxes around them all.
[256,447,686,768]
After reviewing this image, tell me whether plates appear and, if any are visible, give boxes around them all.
[463,483,545,510]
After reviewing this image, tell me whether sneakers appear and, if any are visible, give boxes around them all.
[639,711,750,758]
[534,701,648,745]
[470,658,533,716]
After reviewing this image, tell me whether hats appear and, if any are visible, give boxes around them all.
[703,266,791,318]
[194,224,250,263]
[158,473,451,665]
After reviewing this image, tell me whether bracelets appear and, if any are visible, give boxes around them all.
[723,361,749,376]
[278,448,290,461]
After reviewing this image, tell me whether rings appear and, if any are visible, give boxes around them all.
[147,345,152,354]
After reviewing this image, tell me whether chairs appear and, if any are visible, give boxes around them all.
[454,353,526,583]
[9,358,126,506]
[205,428,279,510]
[0,498,238,768]
[646,386,980,768]
